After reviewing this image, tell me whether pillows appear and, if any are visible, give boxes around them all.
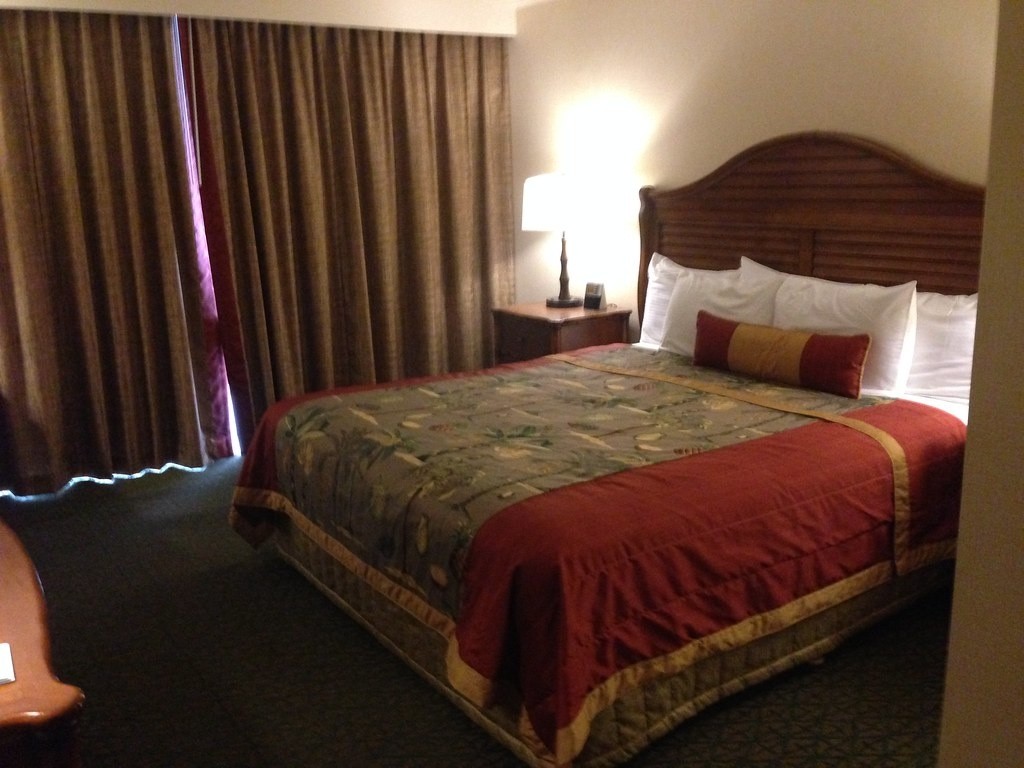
[636,249,978,409]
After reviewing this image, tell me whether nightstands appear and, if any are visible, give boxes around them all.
[489,300,632,364]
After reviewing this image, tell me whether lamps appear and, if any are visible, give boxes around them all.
[521,172,603,307]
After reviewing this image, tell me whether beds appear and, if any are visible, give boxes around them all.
[241,126,985,767]
